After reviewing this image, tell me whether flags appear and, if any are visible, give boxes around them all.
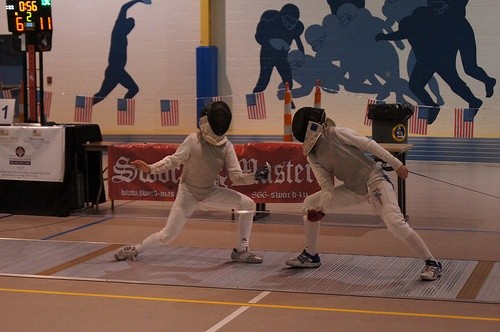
[246,93,266,120]
[201,96,220,106]
[117,98,135,125]
[0,90,16,117]
[74,96,93,122]
[35,91,52,117]
[363,98,386,126]
[454,108,474,138]
[408,106,428,135]
[160,99,179,126]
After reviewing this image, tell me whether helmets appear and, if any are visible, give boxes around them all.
[199,100,232,136]
[291,107,326,142]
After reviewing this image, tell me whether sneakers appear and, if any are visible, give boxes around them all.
[230,247,263,264]
[285,248,321,268]
[114,245,137,261]
[419,259,443,281]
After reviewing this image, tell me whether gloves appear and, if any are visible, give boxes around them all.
[255,164,270,180]
[307,208,326,222]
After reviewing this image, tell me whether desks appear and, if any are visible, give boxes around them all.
[0,124,413,222]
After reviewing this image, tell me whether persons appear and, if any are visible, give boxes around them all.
[285,107,442,280]
[114,100,272,264]
